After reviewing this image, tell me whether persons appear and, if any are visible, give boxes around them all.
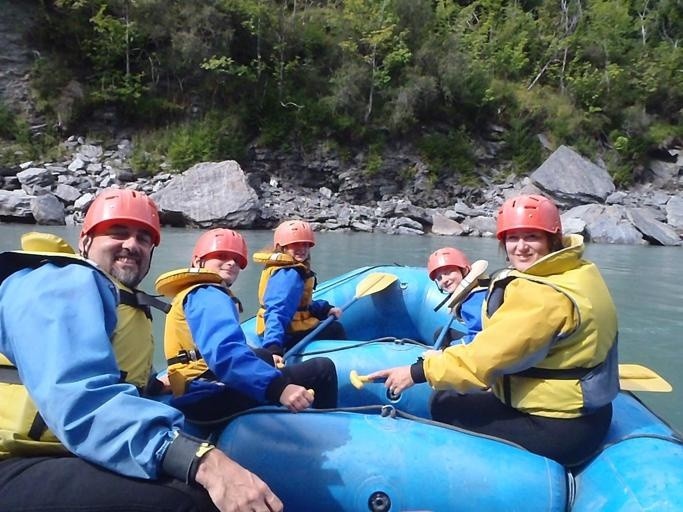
[153,228,339,412]
[253,220,346,369]
[0,188,285,512]
[367,195,621,465]
[427,247,491,350]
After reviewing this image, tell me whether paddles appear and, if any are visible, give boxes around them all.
[349,362,673,393]
[281,273,397,365]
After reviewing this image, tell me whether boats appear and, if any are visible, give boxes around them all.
[155,263,682,512]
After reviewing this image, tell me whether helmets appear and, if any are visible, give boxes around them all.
[426,246,472,282]
[495,192,564,244]
[191,227,248,271]
[77,187,162,248]
[273,220,316,252]
